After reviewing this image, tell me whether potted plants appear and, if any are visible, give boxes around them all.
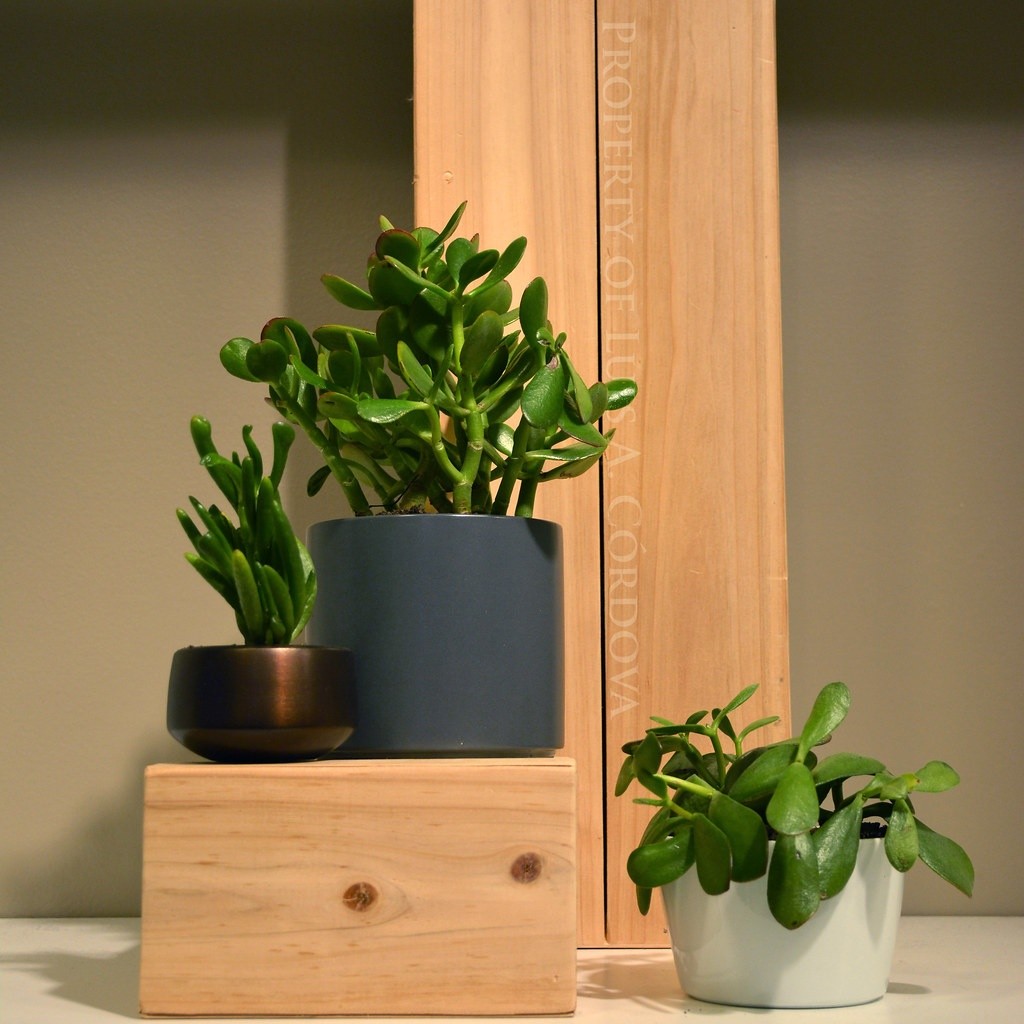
[220,200,635,755]
[165,415,357,763]
[614,681,975,1010]
